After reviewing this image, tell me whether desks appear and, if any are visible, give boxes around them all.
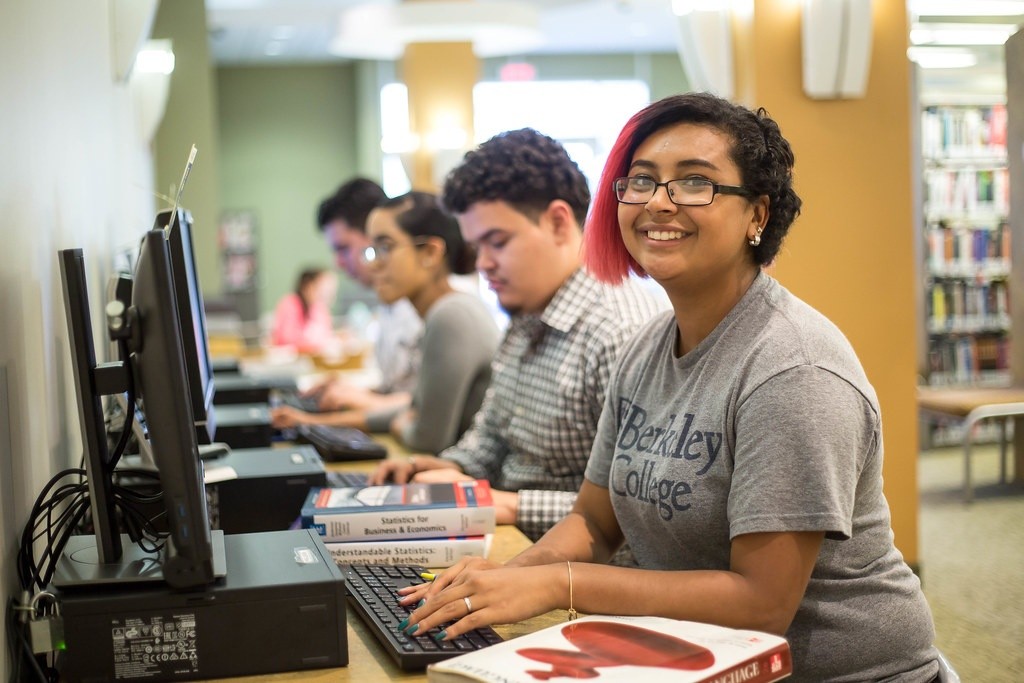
[202,390,585,683]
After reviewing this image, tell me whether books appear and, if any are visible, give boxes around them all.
[921,105,1016,445]
[302,477,495,569]
[427,612,791,683]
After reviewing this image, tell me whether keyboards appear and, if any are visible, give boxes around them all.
[335,561,506,673]
[290,387,329,414]
[298,423,388,464]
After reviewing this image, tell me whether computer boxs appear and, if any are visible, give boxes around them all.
[43,358,350,683]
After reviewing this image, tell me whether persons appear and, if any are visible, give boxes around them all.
[268,177,505,455]
[367,127,674,544]
[397,92,939,683]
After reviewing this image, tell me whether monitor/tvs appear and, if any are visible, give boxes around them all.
[132,228,216,586]
[152,210,218,444]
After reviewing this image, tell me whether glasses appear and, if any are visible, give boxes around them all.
[363,234,431,262]
[612,177,757,206]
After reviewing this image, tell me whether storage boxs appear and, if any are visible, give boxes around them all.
[300,479,496,543]
[322,534,493,568]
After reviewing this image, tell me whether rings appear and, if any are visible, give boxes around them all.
[464,597,471,613]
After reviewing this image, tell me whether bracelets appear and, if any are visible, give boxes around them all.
[568,561,577,621]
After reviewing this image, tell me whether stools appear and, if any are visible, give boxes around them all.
[920,386,1024,507]
